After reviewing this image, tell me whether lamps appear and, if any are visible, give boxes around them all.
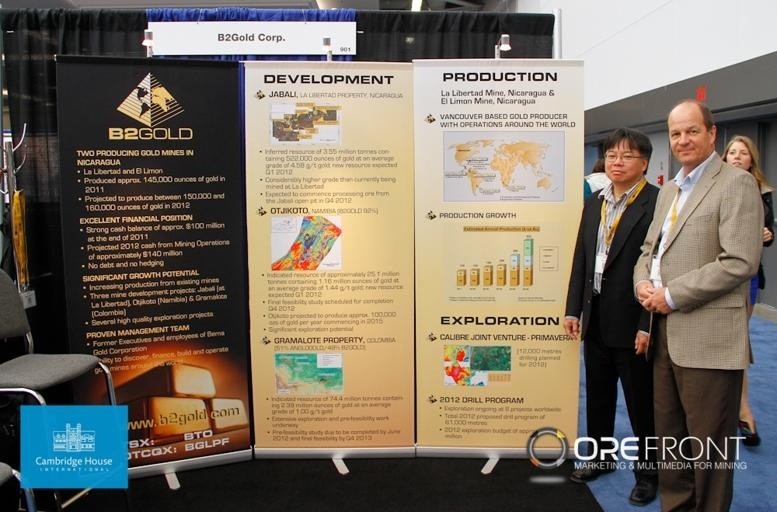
[142,31,153,57]
[495,34,512,58]
[322,37,332,61]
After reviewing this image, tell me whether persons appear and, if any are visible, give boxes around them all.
[562,125,661,506]
[720,133,775,448]
[584,159,605,203]
[632,98,765,511]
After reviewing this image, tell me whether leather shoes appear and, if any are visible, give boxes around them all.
[571,459,617,484]
[630,474,658,507]
[736,420,762,447]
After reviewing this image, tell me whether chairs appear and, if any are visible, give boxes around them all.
[0,461,37,512]
[0,268,119,512]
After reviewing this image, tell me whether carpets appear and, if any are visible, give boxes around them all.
[574,316,777,512]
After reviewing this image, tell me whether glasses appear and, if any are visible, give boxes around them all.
[605,152,645,161]
